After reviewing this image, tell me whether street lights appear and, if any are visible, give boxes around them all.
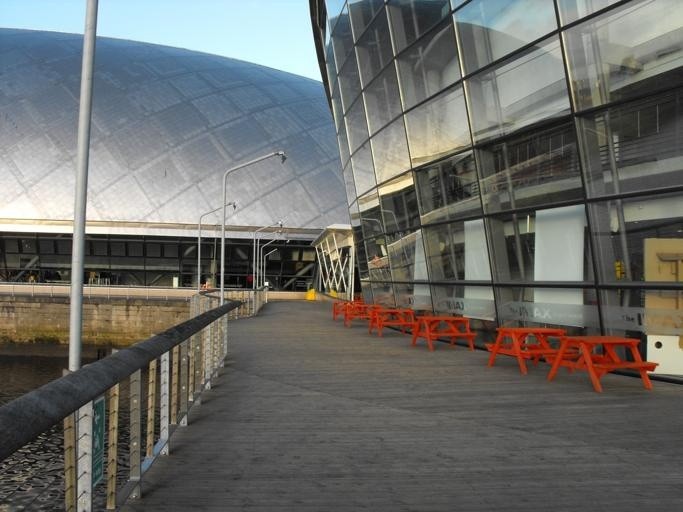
[219,150,286,306]
[251,221,289,290]
[197,202,235,292]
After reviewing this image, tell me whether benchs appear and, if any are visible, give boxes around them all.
[332,300,477,351]
[547,336,658,392]
[484,327,573,375]
